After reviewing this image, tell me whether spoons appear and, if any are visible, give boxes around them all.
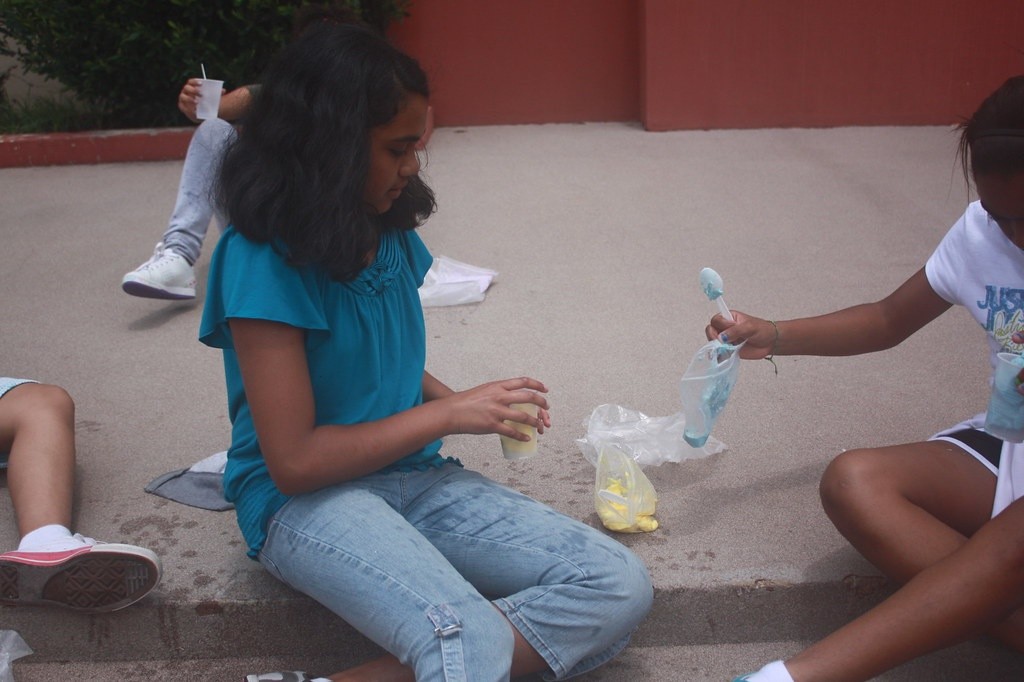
[699,268,734,321]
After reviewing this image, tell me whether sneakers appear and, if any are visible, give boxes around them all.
[246,671,314,682]
[0,532,163,612]
[123,241,197,299]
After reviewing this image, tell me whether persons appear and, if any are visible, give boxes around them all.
[706,75,1024,682]
[122,18,656,682]
[0,377,161,616]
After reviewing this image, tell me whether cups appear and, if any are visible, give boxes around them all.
[193,78,224,119]
[984,352,1024,443]
[500,388,539,459]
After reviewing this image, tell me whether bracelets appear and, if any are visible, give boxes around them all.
[757,319,779,375]
